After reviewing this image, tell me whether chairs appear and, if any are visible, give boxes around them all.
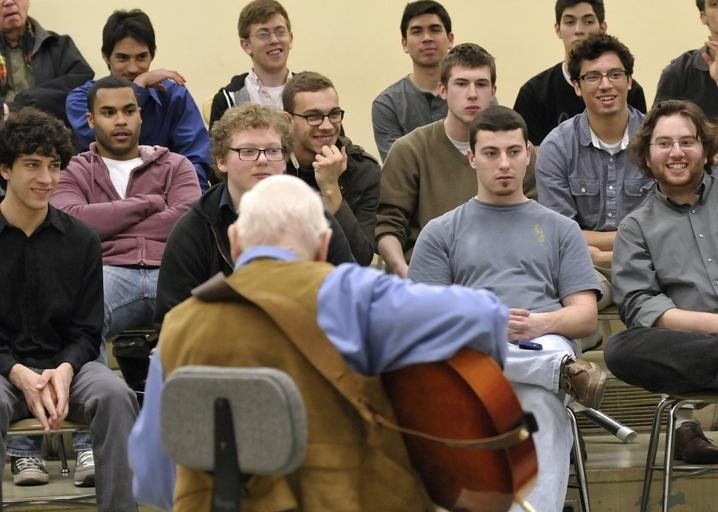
[161,365,307,510]
[551,377,717,507]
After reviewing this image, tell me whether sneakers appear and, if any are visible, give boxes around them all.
[74,449,96,487]
[11,454,50,486]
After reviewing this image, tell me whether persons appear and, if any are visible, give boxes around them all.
[513,0,647,146]
[280,73,382,265]
[407,107,606,510]
[209,0,298,134]
[1,107,139,511]
[603,98,718,399]
[8,424,54,487]
[48,77,202,487]
[127,174,510,510]
[534,32,718,463]
[0,1,95,195]
[372,1,499,162]
[65,7,213,198]
[154,106,358,327]
[375,44,536,280]
[651,0,718,131]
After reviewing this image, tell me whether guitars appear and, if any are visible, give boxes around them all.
[381,348,539,511]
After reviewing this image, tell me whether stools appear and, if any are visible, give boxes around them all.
[2,419,96,506]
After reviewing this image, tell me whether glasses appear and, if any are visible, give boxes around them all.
[249,26,289,41]
[652,136,702,149]
[229,145,287,162]
[577,70,629,84]
[291,110,344,126]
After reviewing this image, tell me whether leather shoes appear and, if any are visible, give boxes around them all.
[560,354,607,411]
[674,420,717,465]
